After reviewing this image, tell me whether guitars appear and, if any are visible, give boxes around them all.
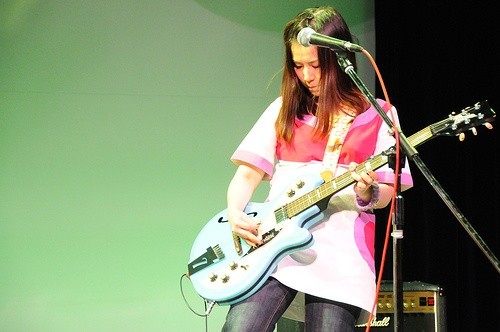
[188,99,496,306]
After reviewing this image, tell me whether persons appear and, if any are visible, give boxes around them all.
[221,6,414,332]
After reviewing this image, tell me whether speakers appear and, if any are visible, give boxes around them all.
[354,281,447,332]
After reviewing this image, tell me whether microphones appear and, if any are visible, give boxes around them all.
[296,28,363,52]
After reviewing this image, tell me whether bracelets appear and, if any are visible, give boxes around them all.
[355,179,380,212]
